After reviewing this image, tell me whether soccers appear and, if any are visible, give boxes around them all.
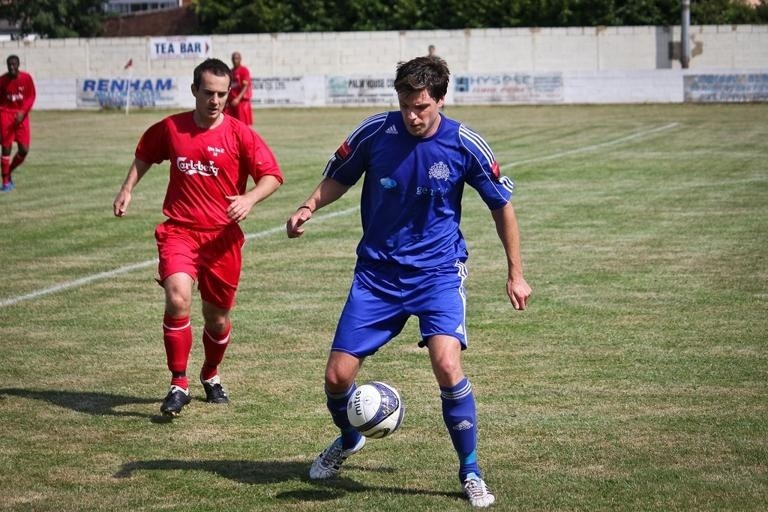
[345,381,403,438]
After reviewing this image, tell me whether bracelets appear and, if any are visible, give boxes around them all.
[297,205,313,212]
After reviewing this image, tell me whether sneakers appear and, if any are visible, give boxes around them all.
[1,175,15,190]
[310,437,366,479]
[160,385,192,414]
[464,477,495,508]
[200,372,229,404]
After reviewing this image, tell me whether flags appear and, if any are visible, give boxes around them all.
[124,58,133,70]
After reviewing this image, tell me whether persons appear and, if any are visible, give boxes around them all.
[224,50,253,129]
[113,56,283,419]
[286,53,532,508]
[0,54,36,193]
